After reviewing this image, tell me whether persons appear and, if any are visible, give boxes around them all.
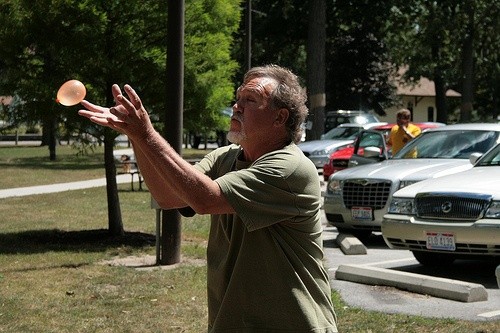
[383,109,421,157]
[79,63,338,333]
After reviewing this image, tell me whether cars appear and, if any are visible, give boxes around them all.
[323,122,500,241]
[326,111,381,134]
[381,140,500,270]
[298,121,386,167]
[323,123,446,181]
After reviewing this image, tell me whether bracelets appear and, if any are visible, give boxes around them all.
[403,131,409,137]
